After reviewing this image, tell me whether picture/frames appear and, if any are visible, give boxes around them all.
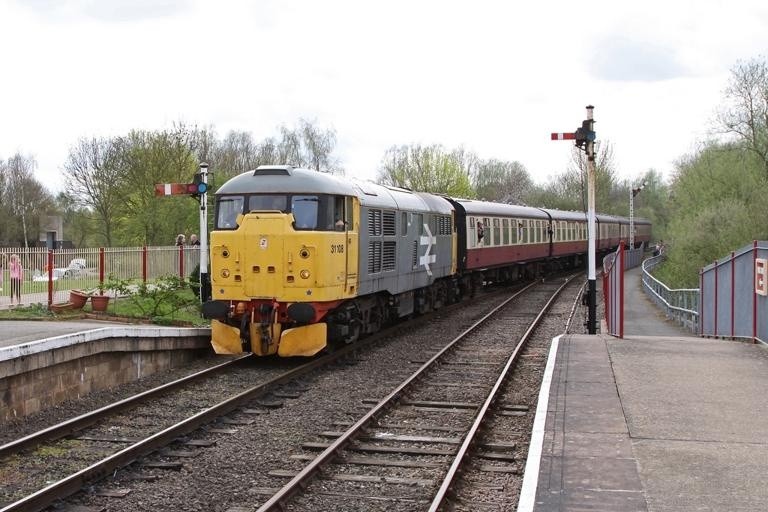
[754,259,768,296]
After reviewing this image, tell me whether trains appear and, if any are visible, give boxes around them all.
[198,163,653,358]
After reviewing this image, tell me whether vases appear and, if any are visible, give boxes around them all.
[49,289,109,314]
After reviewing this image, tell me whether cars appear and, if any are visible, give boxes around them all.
[34,257,97,281]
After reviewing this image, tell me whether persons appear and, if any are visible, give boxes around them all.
[190,234,200,246]
[9,254,24,305]
[175,233,186,246]
